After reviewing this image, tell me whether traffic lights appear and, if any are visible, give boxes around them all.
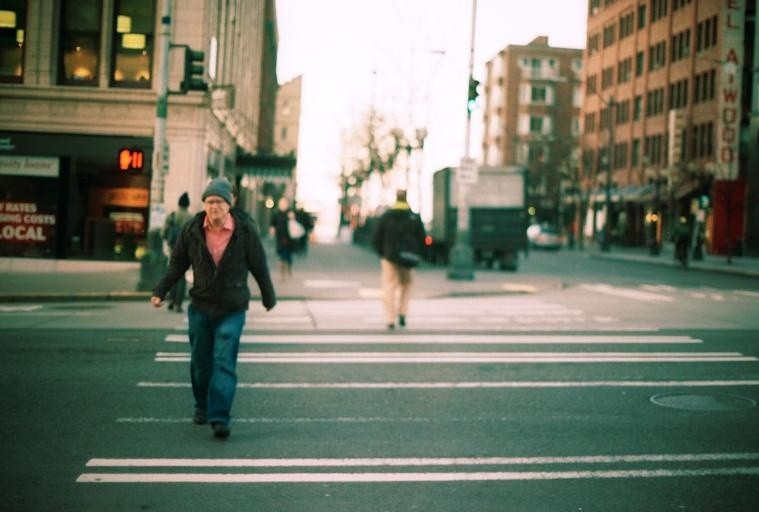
[699,172,712,209]
[182,47,204,91]
[468,78,478,113]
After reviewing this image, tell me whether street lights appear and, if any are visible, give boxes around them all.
[568,78,613,251]
[393,126,426,192]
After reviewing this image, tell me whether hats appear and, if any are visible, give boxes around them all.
[177,192,190,208]
[201,177,234,207]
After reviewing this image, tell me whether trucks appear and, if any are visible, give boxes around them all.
[433,165,527,267]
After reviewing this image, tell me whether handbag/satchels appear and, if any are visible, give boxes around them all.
[400,235,420,266]
[166,227,181,248]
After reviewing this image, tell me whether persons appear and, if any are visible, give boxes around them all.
[371,186,426,329]
[267,191,299,277]
[160,190,194,314]
[151,174,276,440]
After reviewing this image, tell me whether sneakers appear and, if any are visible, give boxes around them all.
[193,407,207,425]
[210,423,230,438]
